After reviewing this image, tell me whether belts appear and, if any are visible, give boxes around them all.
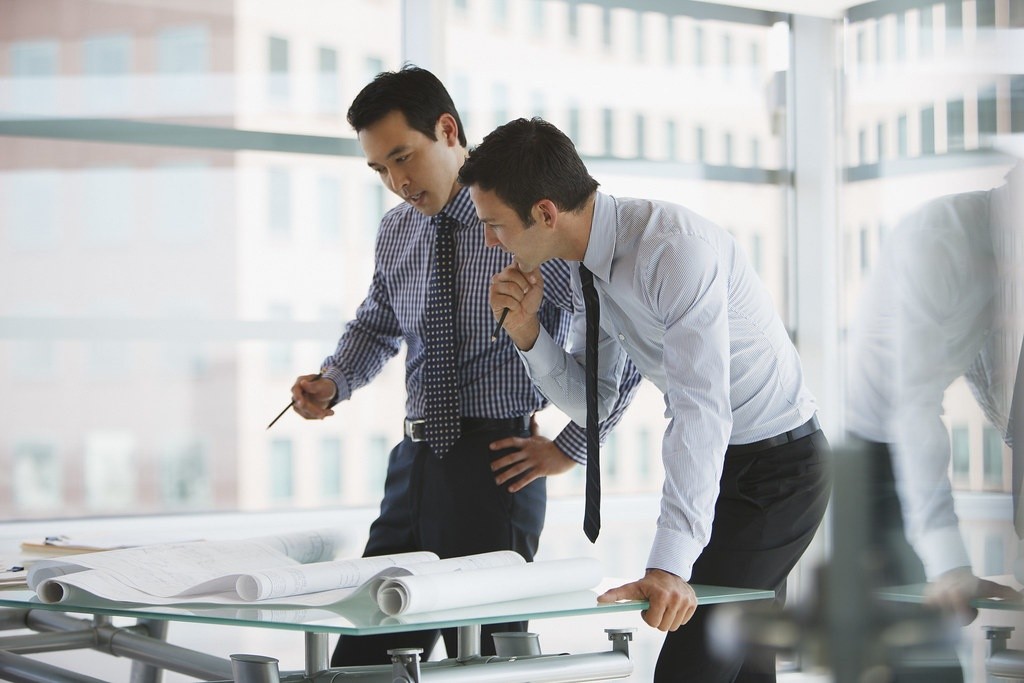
[724,414,821,457]
[402,415,532,444]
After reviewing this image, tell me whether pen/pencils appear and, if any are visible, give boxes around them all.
[264,366,328,430]
[490,308,510,342]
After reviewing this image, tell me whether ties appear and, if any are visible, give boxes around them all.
[424,213,462,462]
[577,263,602,546]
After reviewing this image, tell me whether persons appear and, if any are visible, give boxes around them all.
[457,117,834,683]
[290,63,642,667]
[835,158,1024,683]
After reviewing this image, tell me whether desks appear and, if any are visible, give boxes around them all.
[0,588,775,683]
[873,582,1024,683]
